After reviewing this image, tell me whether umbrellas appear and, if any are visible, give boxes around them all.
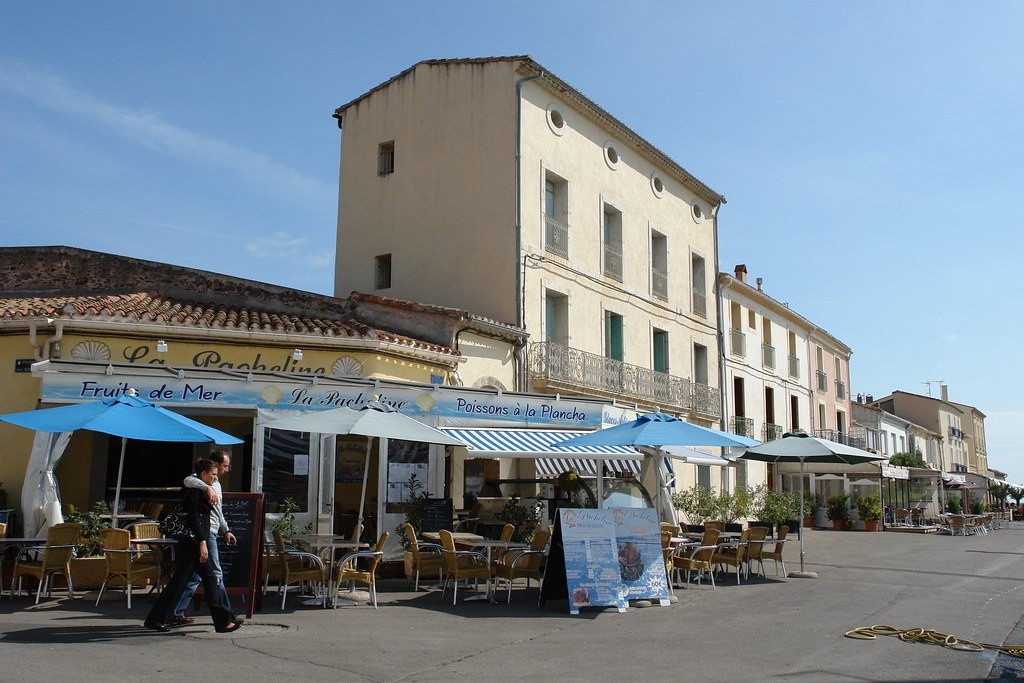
[256,395,467,593]
[721,432,891,572]
[0,388,245,529]
[551,406,762,536]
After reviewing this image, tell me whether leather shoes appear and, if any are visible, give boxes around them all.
[215,623,241,632]
[147,623,170,631]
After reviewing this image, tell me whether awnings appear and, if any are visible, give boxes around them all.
[645,445,739,467]
[436,427,677,477]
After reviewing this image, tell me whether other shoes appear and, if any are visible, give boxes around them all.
[172,616,193,624]
[233,618,244,624]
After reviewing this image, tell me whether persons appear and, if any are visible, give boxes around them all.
[144,450,245,634]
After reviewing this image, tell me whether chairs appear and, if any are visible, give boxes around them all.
[0,520,789,610]
[885,506,994,537]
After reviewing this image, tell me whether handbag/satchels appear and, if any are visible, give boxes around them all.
[157,504,203,543]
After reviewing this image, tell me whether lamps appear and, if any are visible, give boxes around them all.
[890,478,895,482]
[293,348,303,361]
[157,340,168,353]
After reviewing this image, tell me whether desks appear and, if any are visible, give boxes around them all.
[422,532,483,587]
[720,531,770,578]
[301,539,370,608]
[282,534,344,599]
[455,539,528,605]
[130,538,178,604]
[679,532,732,582]
[0,538,48,605]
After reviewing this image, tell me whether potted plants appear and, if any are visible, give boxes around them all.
[824,488,852,530]
[798,490,825,527]
[775,488,812,534]
[854,494,884,532]
[744,480,784,539]
[670,481,720,542]
[712,485,757,533]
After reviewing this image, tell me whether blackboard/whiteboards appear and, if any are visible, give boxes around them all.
[193,491,266,595]
[420,498,454,551]
